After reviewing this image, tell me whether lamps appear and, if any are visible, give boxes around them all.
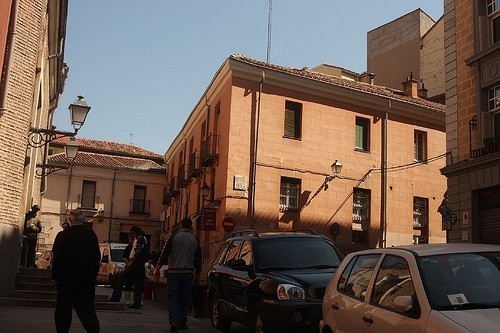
[34,136,80,179]
[324,159,343,183]
[25,95,91,150]
[201,180,221,205]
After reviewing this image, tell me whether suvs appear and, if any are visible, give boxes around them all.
[207,226,349,333]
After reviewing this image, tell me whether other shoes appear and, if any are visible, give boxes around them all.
[169,326,177,333]
[176,325,188,330]
[128,305,141,309]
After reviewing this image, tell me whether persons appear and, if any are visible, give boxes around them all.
[21,205,42,268]
[153,219,201,333]
[62,222,70,230]
[51,209,101,333]
[109,226,152,309]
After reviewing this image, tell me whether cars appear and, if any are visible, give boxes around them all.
[319,243,500,333]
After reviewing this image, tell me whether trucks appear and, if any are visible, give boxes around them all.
[96,243,129,285]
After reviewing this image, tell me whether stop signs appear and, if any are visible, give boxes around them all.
[221,217,235,233]
